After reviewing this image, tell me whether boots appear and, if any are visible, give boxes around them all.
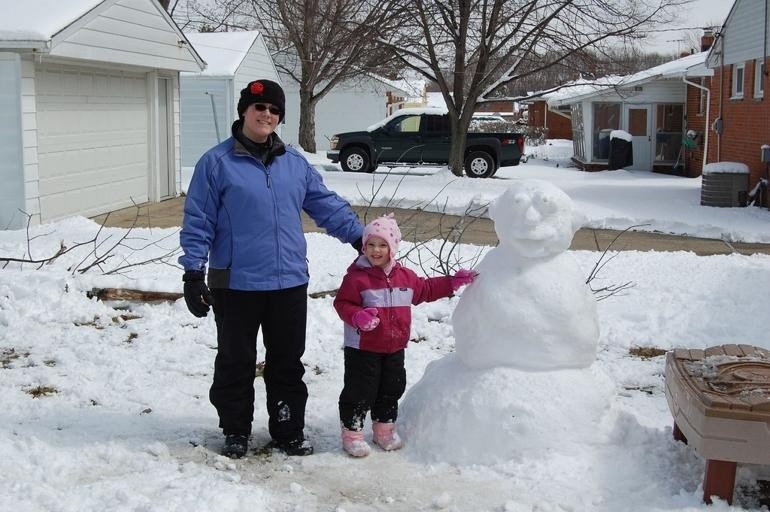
[371,420,402,451]
[342,424,371,457]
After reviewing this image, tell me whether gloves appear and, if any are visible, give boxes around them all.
[183,269,215,318]
[451,269,477,291]
[354,307,381,333]
[352,236,363,255]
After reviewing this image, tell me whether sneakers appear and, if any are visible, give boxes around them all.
[271,437,314,457]
[221,432,250,459]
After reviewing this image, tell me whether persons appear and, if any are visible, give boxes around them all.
[331,210,480,457]
[174,75,368,459]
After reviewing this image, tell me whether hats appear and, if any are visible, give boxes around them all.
[238,79,285,124]
[361,212,402,277]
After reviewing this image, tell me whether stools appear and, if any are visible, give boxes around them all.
[663,344,770,506]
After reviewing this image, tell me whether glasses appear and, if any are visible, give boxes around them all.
[250,102,281,115]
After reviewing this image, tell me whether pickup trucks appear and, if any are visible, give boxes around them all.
[327,108,524,178]
